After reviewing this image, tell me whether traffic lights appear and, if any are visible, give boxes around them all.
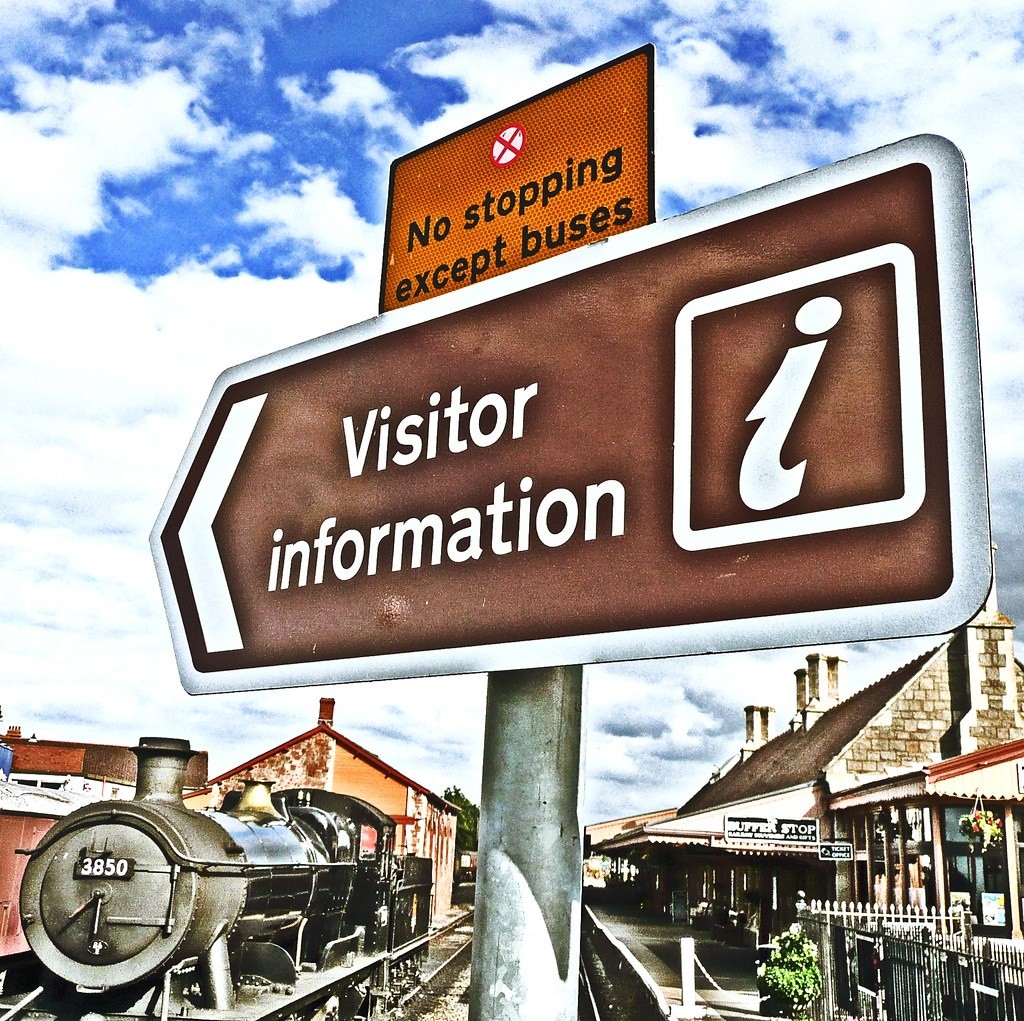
[817,843,854,862]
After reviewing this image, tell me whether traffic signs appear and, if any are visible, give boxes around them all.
[147,128,996,698]
[724,813,822,845]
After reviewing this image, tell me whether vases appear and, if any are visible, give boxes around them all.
[759,996,808,1020]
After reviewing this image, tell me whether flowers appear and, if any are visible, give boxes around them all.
[958,809,1007,855]
[754,926,823,1009]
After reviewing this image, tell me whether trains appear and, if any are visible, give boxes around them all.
[0,733,433,1021]
